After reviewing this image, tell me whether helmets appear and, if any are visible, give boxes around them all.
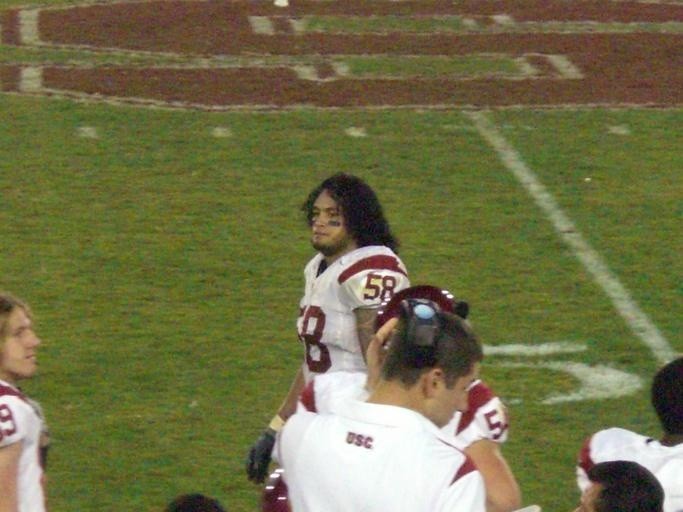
[374,286,468,351]
[262,468,291,512]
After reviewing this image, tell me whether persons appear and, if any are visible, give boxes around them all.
[165,495,223,511]
[249,173,410,484]
[272,301,488,512]
[574,356,683,511]
[261,286,521,512]
[574,458,666,511]
[0,293,50,512]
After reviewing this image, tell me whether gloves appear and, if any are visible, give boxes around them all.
[246,433,276,485]
